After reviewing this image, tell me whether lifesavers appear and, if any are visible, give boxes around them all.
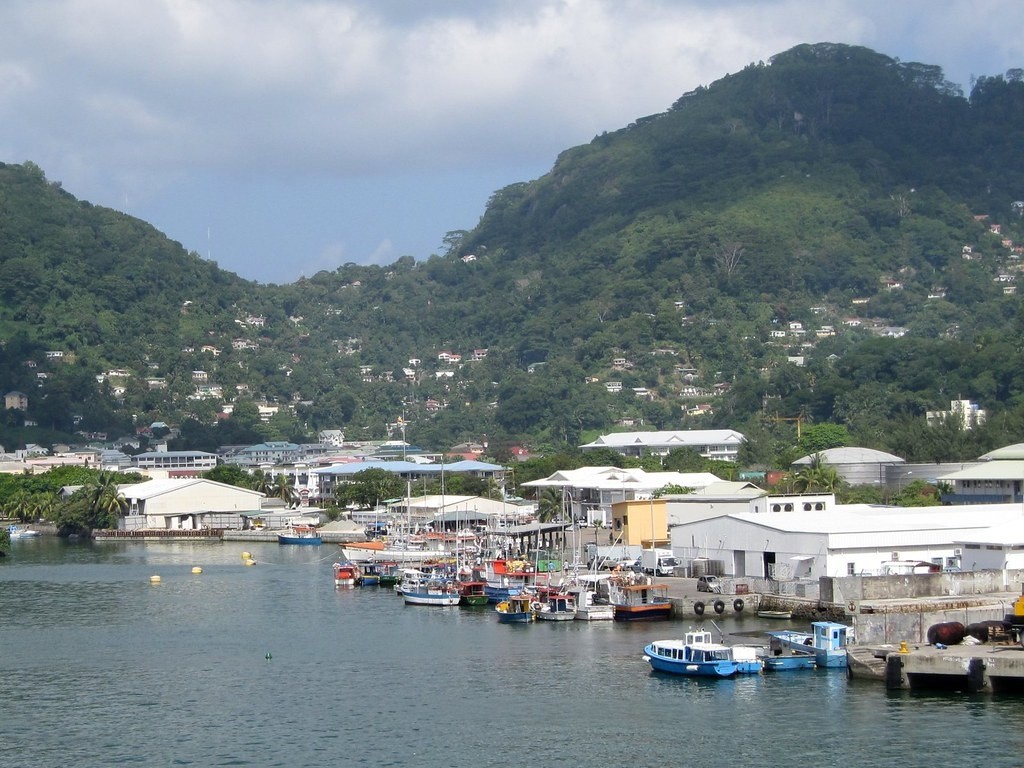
[733,598,744,611]
[694,602,705,615]
[714,600,725,614]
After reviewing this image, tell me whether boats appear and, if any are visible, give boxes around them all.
[277,521,324,546]
[643,619,764,677]
[332,461,672,623]
[4,523,43,538]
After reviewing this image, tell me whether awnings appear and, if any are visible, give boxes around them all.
[790,556,814,560]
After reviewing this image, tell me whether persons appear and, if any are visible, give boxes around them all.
[476,557,481,566]
[256,517,262,524]
[497,554,500,560]
[609,562,614,573]
[549,561,555,576]
[564,559,569,575]
